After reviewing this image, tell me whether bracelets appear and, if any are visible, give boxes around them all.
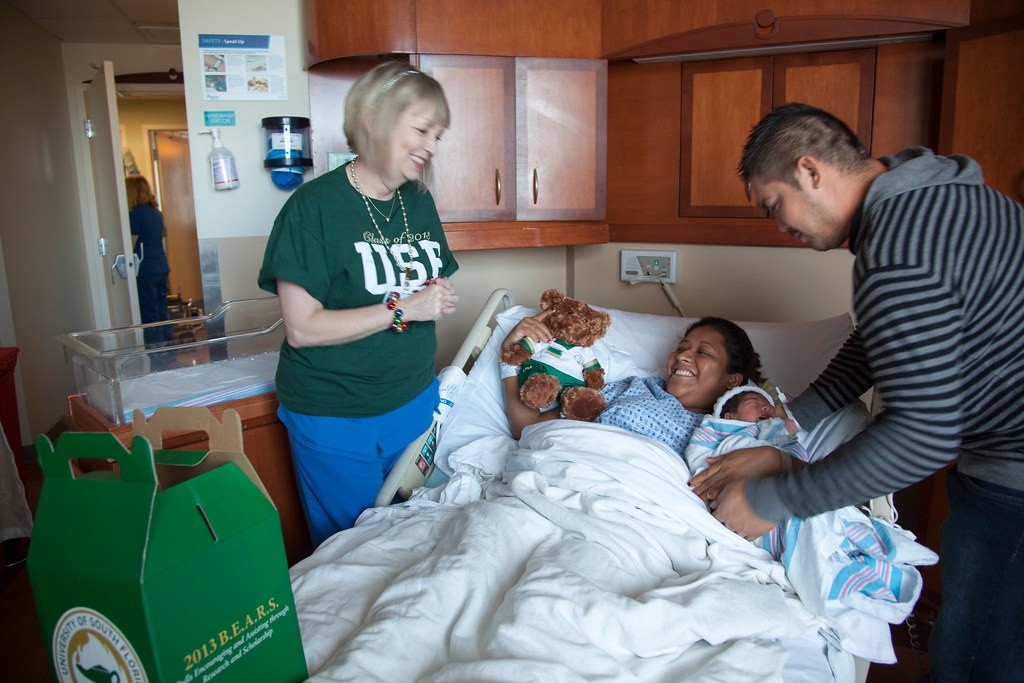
[388,295,407,333]
[501,362,520,380]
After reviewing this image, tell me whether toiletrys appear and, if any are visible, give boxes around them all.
[197,127,240,191]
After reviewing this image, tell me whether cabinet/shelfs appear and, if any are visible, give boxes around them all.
[64,390,310,569]
[615,0,973,250]
[301,0,609,252]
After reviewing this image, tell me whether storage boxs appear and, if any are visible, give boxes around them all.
[27,405,310,683]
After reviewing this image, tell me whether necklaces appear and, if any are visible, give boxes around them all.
[351,157,413,272]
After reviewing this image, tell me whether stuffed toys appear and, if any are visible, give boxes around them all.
[502,290,612,421]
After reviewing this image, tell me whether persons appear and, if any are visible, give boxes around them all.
[259,61,459,552]
[686,386,937,624]
[126,175,179,370]
[710,102,1024,683]
[500,308,813,504]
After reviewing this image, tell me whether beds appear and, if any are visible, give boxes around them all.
[286,286,894,683]
